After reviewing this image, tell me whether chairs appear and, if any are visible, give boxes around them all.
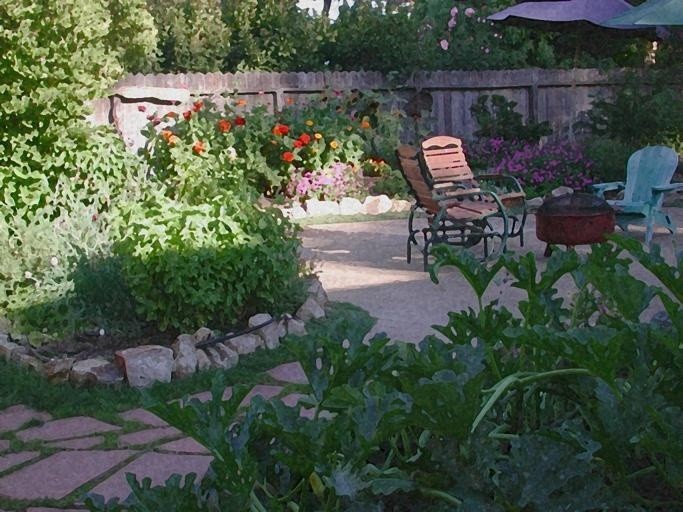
[395,145,508,272]
[593,145,683,247]
[421,136,527,248]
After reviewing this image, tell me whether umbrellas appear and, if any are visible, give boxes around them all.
[604,0,683,30]
[485,0,670,138]
